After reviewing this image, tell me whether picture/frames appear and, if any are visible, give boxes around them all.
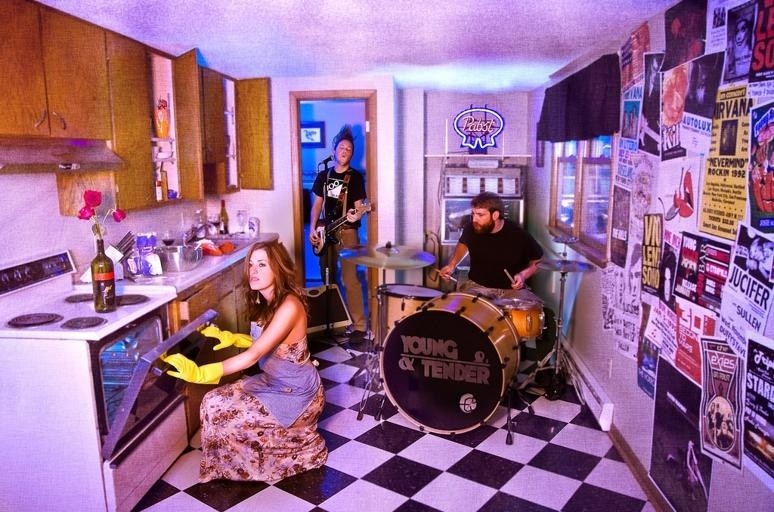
[301,122,325,149]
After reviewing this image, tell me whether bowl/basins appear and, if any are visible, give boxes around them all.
[155,246,202,272]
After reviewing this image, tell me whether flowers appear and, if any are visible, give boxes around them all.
[76,189,126,239]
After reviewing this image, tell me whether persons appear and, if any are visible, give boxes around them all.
[160,239,327,483]
[440,192,556,386]
[311,125,365,343]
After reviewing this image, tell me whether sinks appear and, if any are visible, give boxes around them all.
[207,237,255,253]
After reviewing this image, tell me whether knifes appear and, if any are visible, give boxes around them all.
[114,231,136,278]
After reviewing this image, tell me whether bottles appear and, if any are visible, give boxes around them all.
[90,237,115,313]
[219,198,229,234]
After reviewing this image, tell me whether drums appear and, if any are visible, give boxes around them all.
[494,298,546,337]
[377,294,522,437]
[376,285,444,348]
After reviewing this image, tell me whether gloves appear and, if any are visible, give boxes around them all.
[196,323,252,350]
[163,353,223,386]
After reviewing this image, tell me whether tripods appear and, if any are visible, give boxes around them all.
[517,273,586,406]
[307,164,356,360]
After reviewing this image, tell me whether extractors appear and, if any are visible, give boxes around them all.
[0,139,124,172]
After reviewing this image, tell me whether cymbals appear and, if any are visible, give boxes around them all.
[536,260,597,273]
[339,245,437,270]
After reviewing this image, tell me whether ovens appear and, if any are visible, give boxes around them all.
[90,309,218,460]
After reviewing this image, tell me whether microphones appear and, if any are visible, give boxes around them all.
[318,154,335,165]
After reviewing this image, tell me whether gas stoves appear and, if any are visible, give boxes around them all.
[0,249,167,332]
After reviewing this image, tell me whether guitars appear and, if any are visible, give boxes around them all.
[313,201,372,257]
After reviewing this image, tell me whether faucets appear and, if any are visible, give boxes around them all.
[186,225,211,243]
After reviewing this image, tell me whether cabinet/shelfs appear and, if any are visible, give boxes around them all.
[166,258,251,436]
[198,66,274,197]
[55,17,205,217]
[1,0,111,141]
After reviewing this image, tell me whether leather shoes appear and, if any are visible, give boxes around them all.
[349,330,367,343]
[534,368,565,390]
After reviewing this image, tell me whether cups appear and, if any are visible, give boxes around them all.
[249,217,260,238]
[237,210,246,233]
[162,231,175,252]
[212,214,222,239]
